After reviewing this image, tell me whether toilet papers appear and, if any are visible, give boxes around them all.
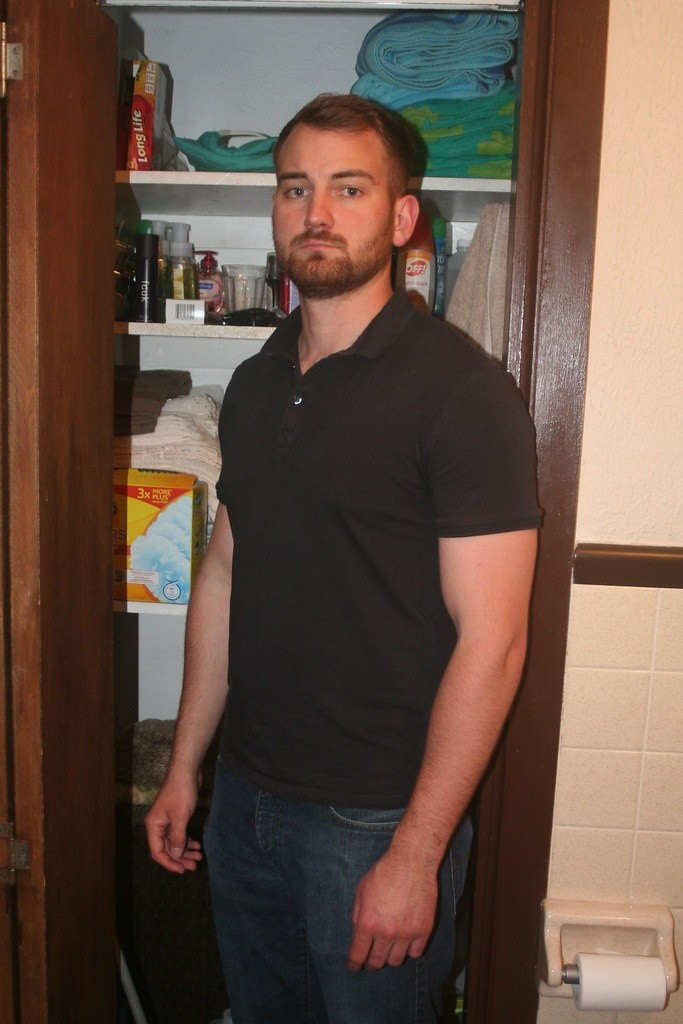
[576,951,673,1011]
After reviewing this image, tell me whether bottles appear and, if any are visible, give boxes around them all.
[396,192,447,319]
[116,221,197,325]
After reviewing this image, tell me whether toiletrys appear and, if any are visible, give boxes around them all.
[136,215,225,320]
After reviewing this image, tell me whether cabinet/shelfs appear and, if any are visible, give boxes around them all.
[111,162,517,892]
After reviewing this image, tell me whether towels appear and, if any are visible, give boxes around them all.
[443,203,511,361]
[349,6,522,179]
[112,369,223,488]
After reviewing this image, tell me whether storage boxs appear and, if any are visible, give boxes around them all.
[125,468,207,604]
[125,59,167,171]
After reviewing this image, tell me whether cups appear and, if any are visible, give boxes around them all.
[222,264,267,326]
[266,252,291,318]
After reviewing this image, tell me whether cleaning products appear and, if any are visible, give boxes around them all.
[395,213,472,323]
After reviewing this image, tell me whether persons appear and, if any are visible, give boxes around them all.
[146,94,540,1023]
